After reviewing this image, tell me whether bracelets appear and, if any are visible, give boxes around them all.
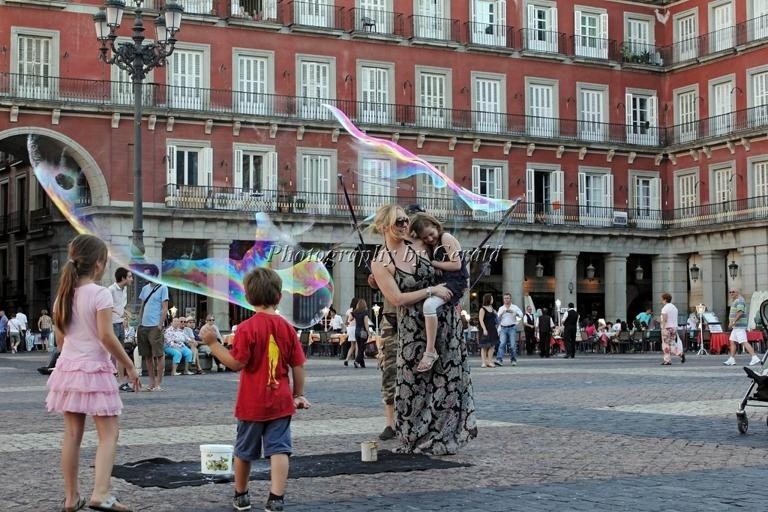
[425,285,432,298]
[293,394,304,398]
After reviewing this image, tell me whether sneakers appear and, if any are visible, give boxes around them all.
[232,493,251,511]
[119,383,134,392]
[380,426,396,440]
[749,357,760,366]
[265,500,284,512]
[723,358,736,366]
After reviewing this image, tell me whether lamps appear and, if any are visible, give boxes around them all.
[482,249,491,278]
[534,250,545,279]
[727,249,739,279]
[568,281,574,295]
[688,252,699,282]
[584,251,595,282]
[634,255,644,283]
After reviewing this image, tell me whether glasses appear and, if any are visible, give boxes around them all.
[181,321,186,323]
[396,218,409,227]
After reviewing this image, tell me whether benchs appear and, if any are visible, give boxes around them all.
[123,353,215,375]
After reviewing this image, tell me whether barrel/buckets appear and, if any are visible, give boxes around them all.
[199,443,235,475]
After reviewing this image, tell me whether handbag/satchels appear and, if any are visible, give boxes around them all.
[360,331,367,339]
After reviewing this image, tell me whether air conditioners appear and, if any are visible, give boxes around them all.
[211,191,239,210]
[292,196,309,213]
[612,209,627,226]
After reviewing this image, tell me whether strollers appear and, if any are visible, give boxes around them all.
[733,296,768,435]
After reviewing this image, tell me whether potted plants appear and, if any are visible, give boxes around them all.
[277,178,289,213]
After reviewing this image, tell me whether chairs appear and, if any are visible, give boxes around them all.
[298,330,381,361]
[577,328,653,352]
[361,15,377,33]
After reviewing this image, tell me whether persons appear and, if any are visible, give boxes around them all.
[138,265,169,392]
[200,268,309,511]
[367,204,426,440]
[0,296,285,375]
[476,290,654,368]
[658,293,687,366]
[410,212,468,372]
[45,235,142,512]
[99,268,132,384]
[722,288,760,365]
[304,294,374,372]
[370,202,479,457]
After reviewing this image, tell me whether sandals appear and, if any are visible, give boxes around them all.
[417,350,439,372]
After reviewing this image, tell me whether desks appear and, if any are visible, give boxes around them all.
[709,330,763,357]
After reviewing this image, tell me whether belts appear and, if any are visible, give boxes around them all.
[501,324,515,328]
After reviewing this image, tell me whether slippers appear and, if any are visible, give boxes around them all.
[63,495,85,511]
[88,495,132,512]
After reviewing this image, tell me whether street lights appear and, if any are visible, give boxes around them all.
[90,1,188,341]
